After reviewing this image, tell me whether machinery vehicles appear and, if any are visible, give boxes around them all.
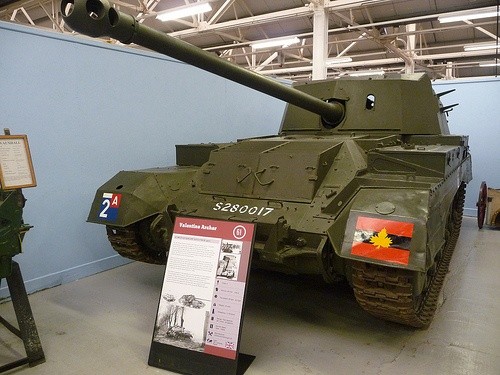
[58,0,476,331]
